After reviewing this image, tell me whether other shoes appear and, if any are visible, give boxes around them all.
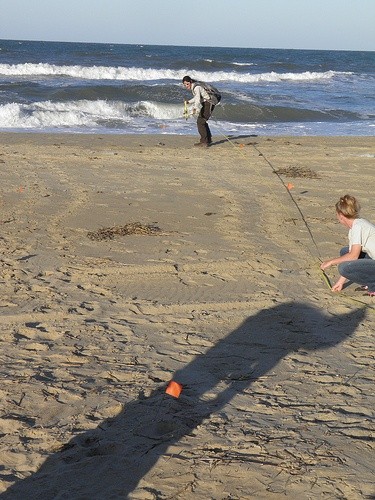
[194,142,208,148]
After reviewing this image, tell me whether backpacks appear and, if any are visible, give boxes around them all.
[192,81,221,106]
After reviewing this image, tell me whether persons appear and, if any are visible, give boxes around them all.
[183,76,215,147]
[321,194,375,296]
